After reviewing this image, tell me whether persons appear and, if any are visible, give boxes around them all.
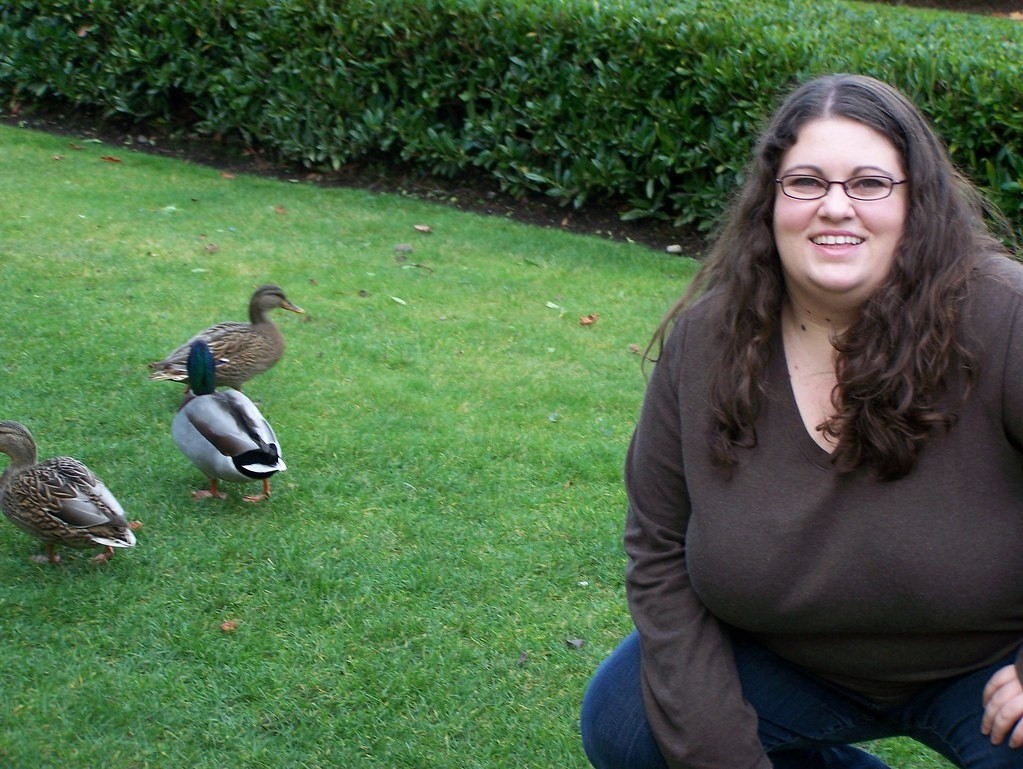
[580,75,1023,769]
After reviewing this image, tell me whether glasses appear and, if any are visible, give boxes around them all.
[774,174,907,200]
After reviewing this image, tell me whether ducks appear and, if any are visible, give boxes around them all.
[147,285,307,411]
[170,339,288,502]
[0,421,140,565]
